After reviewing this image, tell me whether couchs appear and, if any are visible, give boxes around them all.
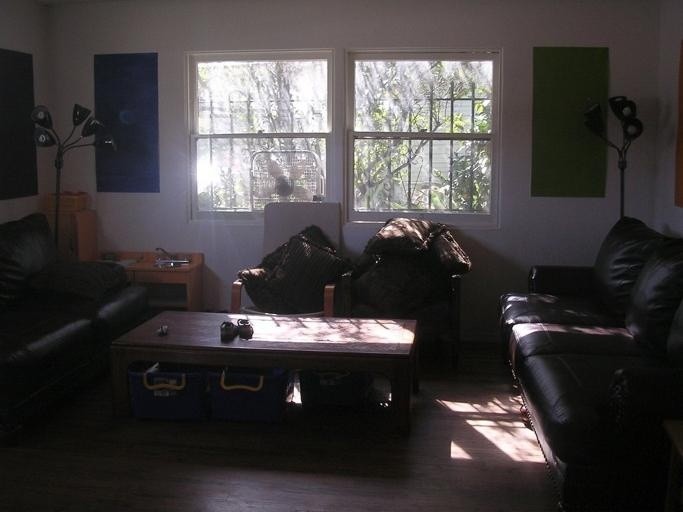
[1,212,148,448]
[497,216,682,512]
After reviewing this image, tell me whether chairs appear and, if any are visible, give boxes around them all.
[231,201,463,373]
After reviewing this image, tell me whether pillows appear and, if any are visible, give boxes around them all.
[237,216,473,312]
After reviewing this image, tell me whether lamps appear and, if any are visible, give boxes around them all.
[583,95,644,218]
[29,101,117,250]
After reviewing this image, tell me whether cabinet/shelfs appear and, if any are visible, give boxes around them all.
[41,192,90,258]
[95,251,205,314]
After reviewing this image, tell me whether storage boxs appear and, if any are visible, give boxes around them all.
[130,364,287,423]
